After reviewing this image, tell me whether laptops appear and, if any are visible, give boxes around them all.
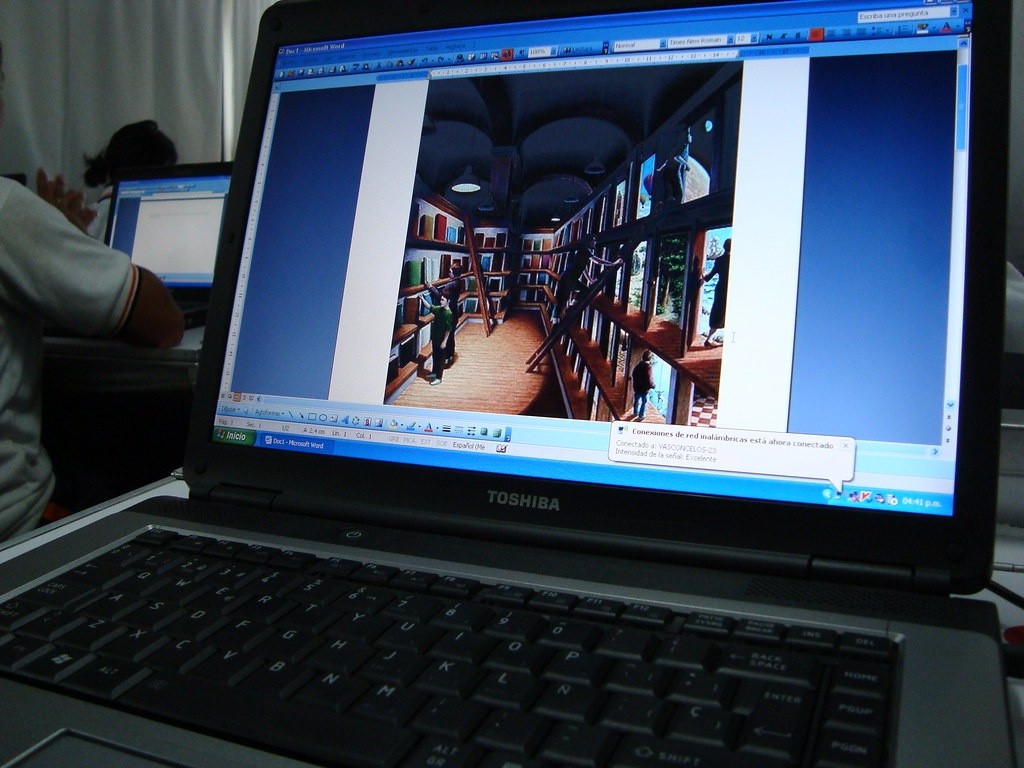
[0,0,1015,768]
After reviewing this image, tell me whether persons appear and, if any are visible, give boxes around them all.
[1,175,184,542]
[36,119,180,242]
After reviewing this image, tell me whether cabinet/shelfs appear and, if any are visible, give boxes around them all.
[382,172,556,403]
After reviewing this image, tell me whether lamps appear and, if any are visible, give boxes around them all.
[550,201,561,222]
[479,173,495,210]
[564,170,579,202]
[584,93,604,173]
[450,116,481,192]
